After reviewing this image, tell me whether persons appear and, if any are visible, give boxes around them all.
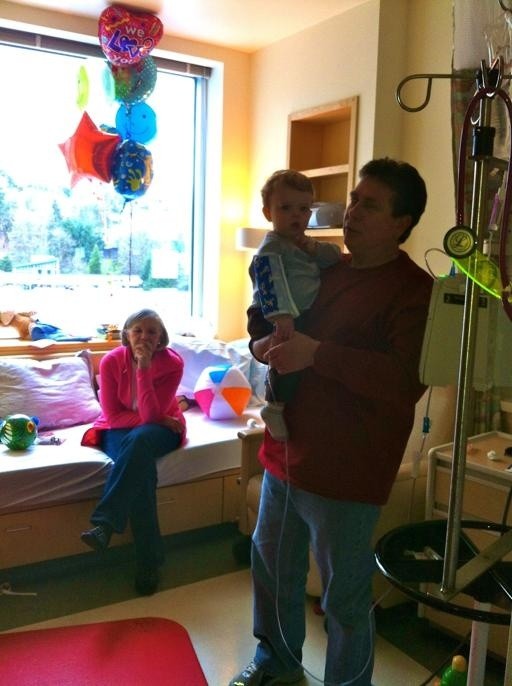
[229,155,435,686]
[244,169,344,445]
[78,308,184,598]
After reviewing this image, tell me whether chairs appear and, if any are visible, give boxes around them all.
[237,381,475,609]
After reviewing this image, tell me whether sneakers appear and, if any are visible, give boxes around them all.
[227,656,302,686]
[132,560,158,598]
[80,524,111,553]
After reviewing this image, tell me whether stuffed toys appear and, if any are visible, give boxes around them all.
[0,303,41,341]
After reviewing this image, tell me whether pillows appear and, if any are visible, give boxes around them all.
[165,336,271,410]
[0,349,103,437]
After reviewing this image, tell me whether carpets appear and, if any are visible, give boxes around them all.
[1,615,206,686]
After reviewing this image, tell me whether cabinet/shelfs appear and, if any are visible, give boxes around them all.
[417,430,512,663]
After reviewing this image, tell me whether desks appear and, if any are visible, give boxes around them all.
[374,519,512,686]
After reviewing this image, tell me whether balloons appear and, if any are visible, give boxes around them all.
[57,1,165,216]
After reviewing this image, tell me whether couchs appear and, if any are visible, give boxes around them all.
[1,334,269,574]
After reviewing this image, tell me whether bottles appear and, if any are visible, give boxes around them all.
[438,652,469,686]
